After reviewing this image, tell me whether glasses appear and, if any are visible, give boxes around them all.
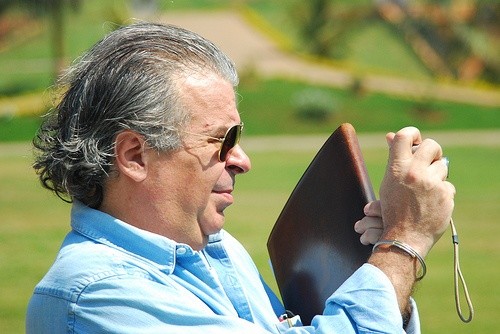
[135,121,245,163]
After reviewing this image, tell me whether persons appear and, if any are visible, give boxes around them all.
[24,18,459,333]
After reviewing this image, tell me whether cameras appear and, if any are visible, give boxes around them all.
[412,143,450,179]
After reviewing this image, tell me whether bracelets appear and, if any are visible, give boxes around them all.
[370,239,429,282]
